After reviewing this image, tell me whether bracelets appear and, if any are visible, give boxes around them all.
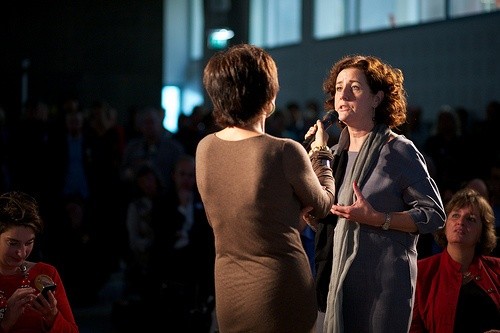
[381,210,392,230]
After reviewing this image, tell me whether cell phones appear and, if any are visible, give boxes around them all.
[31,283,58,308]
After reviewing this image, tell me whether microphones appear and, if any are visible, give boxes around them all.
[302,109,339,147]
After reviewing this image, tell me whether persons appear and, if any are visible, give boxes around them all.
[196,44,336,333]
[0,190,80,333]
[392,99,500,257]
[303,52,446,333]
[0,91,323,333]
[408,187,500,333]
